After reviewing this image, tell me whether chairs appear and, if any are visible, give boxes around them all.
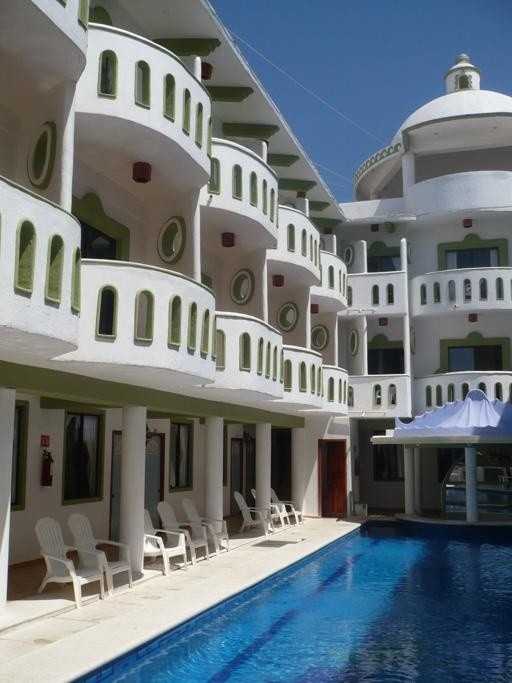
[231,484,305,539]
[31,494,226,609]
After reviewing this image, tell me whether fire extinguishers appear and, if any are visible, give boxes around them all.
[41,450,53,486]
